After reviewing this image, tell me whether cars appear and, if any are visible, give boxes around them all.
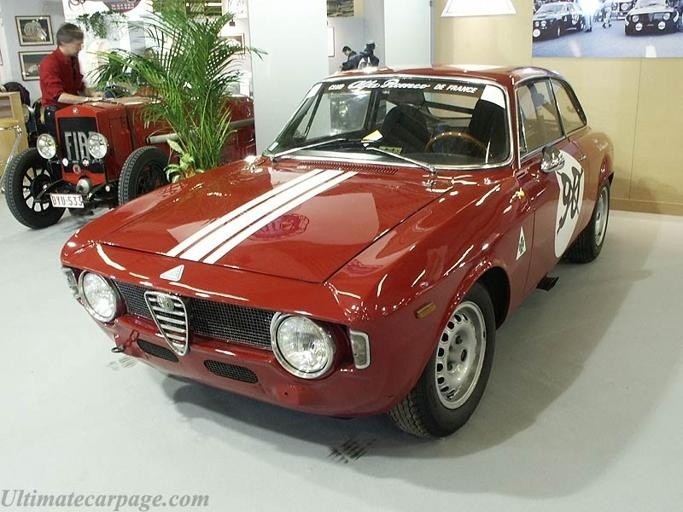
[625,0,680,35]
[532,2,586,39]
[5,52,615,440]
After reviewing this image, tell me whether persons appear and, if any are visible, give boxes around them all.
[601,0,617,28]
[342,45,356,58]
[40,21,106,215]
[341,41,380,71]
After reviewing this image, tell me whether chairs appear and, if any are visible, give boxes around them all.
[375,87,506,159]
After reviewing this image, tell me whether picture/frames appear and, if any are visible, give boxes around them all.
[14,15,54,82]
